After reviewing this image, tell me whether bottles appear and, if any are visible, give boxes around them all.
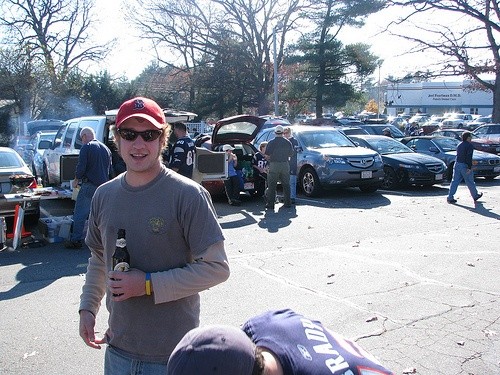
[112,229,131,299]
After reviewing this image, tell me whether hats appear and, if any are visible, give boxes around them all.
[115,96,166,129]
[275,124,284,133]
[223,144,235,151]
[167,323,256,375]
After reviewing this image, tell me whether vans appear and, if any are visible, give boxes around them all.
[42,109,198,185]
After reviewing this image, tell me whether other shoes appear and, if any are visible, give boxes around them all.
[64,240,82,248]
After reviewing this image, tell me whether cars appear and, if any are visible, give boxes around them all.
[399,137,500,185]
[466,117,492,130]
[472,124,500,137]
[23,119,65,184]
[0,147,41,226]
[358,124,405,138]
[191,115,267,200]
[425,129,500,155]
[346,135,447,191]
[390,113,445,126]
[257,115,291,129]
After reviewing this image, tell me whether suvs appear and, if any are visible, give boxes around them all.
[441,114,473,129]
[252,126,384,196]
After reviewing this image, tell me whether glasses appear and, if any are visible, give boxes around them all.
[117,125,168,142]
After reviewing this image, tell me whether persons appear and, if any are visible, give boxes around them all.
[265,123,294,208]
[278,126,299,204]
[252,141,271,203]
[165,306,396,374]
[78,96,230,375]
[162,120,201,179]
[64,126,114,248]
[403,117,432,136]
[446,131,483,203]
[221,143,242,206]
[381,127,394,139]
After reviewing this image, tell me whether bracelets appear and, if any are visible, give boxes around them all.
[145,271,152,296]
